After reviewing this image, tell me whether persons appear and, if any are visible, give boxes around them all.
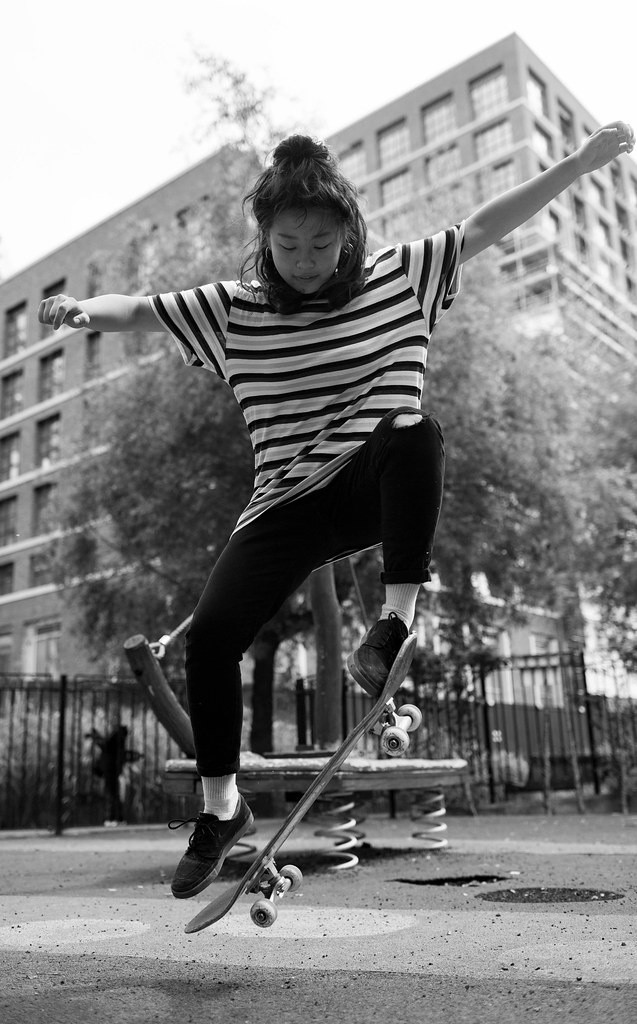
[38,120,637,899]
[92,714,128,827]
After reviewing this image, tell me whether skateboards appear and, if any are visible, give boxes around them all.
[183,630,421,934]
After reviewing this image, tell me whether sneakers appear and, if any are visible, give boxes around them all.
[348,612,409,697]
[168,792,254,898]
